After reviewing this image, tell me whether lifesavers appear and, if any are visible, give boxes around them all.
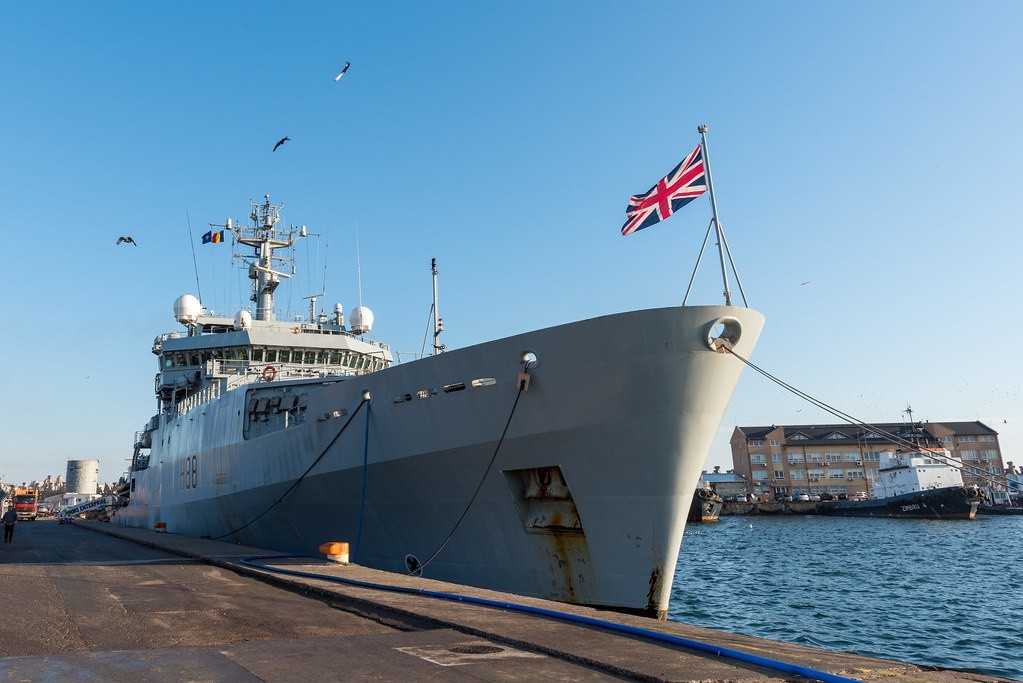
[295,328,300,333]
[263,366,276,382]
[698,490,708,498]
[962,488,986,497]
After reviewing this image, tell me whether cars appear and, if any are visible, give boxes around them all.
[735,494,747,503]
[839,493,848,499]
[782,494,793,501]
[820,492,833,502]
[794,492,809,501]
[855,491,868,501]
[809,493,821,502]
[724,495,734,503]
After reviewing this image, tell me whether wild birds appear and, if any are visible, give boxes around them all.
[117,236,136,247]
[334,61,351,82]
[273,135,291,152]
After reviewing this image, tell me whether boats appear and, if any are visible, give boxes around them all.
[107,124,765,620]
[820,403,986,519]
[686,472,723,521]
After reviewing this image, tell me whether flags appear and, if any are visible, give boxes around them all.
[212,230,225,243]
[619,143,706,234]
[202,230,212,244]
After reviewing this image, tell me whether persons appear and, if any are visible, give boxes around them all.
[0,506,17,543]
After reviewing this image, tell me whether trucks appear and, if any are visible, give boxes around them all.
[13,487,38,520]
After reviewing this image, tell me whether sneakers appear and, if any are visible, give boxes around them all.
[5,540,7,543]
[9,540,11,543]
